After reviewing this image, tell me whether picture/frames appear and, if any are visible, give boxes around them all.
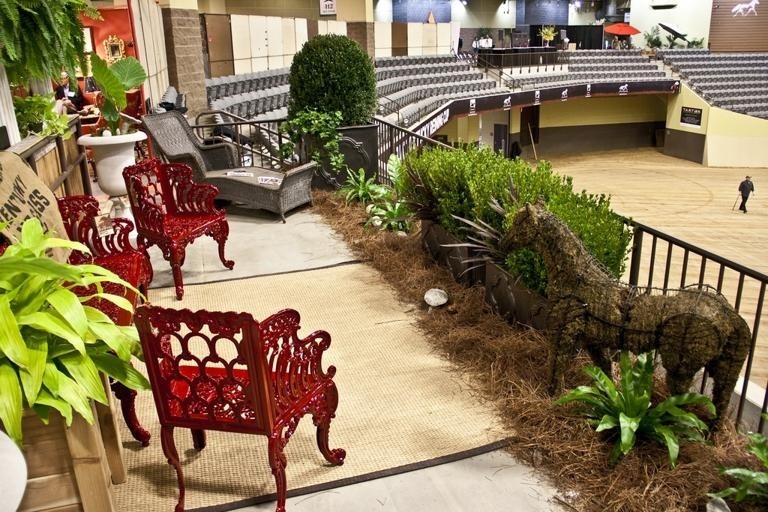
[102,34,126,63]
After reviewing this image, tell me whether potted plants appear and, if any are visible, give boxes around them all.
[536,26,559,47]
[0,217,152,512]
[75,52,147,198]
[465,148,634,330]
[289,35,381,189]
[14,91,69,139]
[397,142,533,288]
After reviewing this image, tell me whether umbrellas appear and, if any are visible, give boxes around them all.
[603,22,641,36]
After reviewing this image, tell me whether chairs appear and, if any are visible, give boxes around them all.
[123,157,235,299]
[80,90,148,183]
[141,110,320,223]
[134,304,346,512]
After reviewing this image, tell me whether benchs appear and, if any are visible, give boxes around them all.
[0,195,153,447]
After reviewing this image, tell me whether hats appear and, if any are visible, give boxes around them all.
[745,176,751,178]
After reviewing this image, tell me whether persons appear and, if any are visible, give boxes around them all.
[738,175,754,212]
[50,72,83,114]
[472,33,493,56]
[612,36,628,49]
